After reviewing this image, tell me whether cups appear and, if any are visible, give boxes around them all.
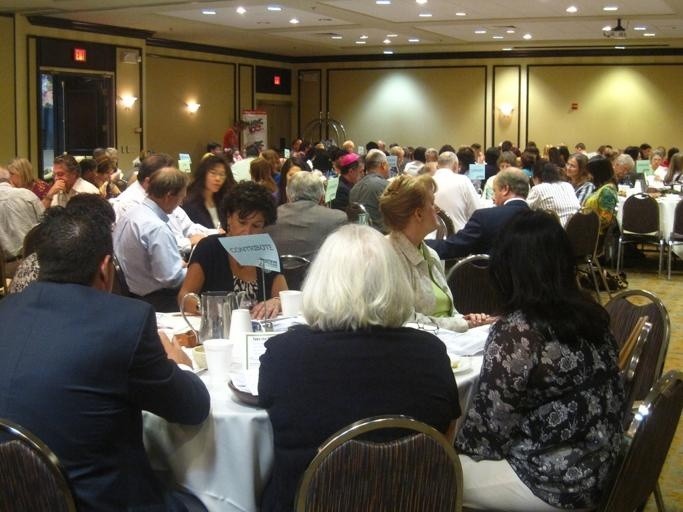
[280,288,301,317]
[206,309,266,381]
[181,287,244,340]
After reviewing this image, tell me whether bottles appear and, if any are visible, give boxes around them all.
[59,190,67,205]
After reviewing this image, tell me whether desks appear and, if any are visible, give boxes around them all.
[408,324,493,414]
[145,308,307,512]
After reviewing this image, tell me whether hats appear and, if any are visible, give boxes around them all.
[338,152,359,165]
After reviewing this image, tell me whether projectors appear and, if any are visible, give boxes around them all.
[602,30,630,40]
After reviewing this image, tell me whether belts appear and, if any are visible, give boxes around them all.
[4,254,23,263]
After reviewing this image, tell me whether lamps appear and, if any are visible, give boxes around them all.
[120,95,138,111]
[187,102,201,115]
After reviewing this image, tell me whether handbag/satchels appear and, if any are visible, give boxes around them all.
[581,267,629,291]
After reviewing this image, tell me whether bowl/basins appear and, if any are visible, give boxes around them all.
[228,380,258,406]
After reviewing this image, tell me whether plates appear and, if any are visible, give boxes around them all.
[449,352,474,376]
[619,190,678,202]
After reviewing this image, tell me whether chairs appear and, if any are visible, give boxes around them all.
[597,289,682,512]
[347,202,374,226]
[433,209,457,241]
[1,416,76,512]
[113,254,132,298]
[564,194,683,301]
[294,415,463,512]
